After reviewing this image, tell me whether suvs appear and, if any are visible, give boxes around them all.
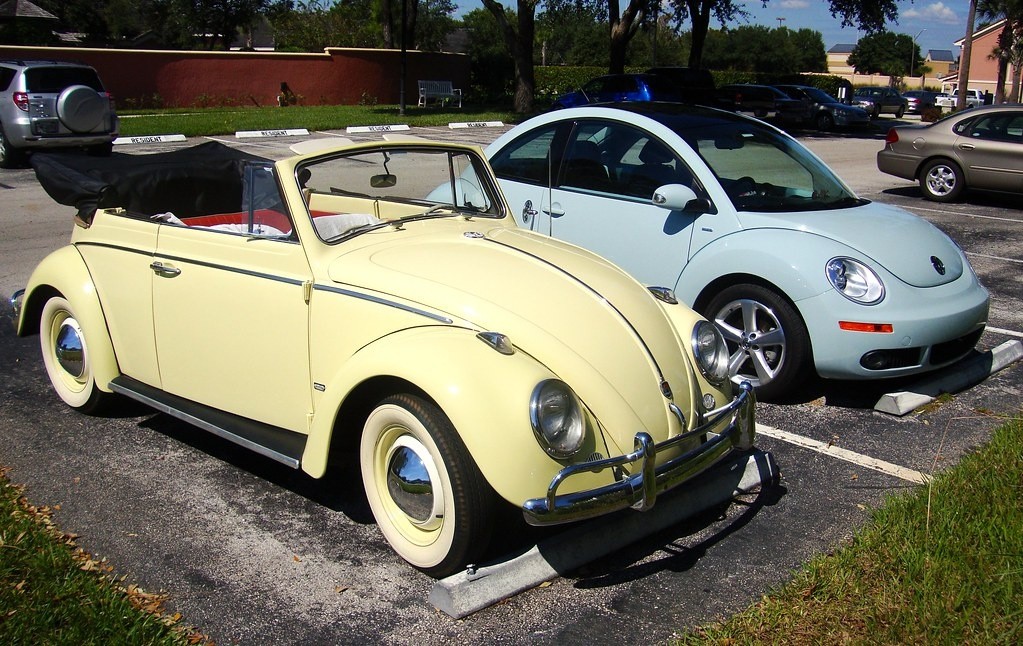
[0,57,120,167]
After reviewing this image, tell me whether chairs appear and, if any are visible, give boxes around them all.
[634,140,677,196]
[565,141,613,194]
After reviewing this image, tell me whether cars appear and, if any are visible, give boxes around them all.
[771,85,870,132]
[423,101,992,400]
[546,73,672,112]
[876,103,1023,203]
[902,91,948,113]
[717,84,810,131]
[646,68,719,109]
[851,87,909,119]
[12,133,756,571]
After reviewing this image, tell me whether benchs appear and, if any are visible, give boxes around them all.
[417,80,462,108]
[180,208,291,234]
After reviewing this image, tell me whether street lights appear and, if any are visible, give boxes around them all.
[776,17,786,27]
[910,29,927,77]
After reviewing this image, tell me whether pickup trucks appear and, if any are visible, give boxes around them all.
[934,88,985,110]
